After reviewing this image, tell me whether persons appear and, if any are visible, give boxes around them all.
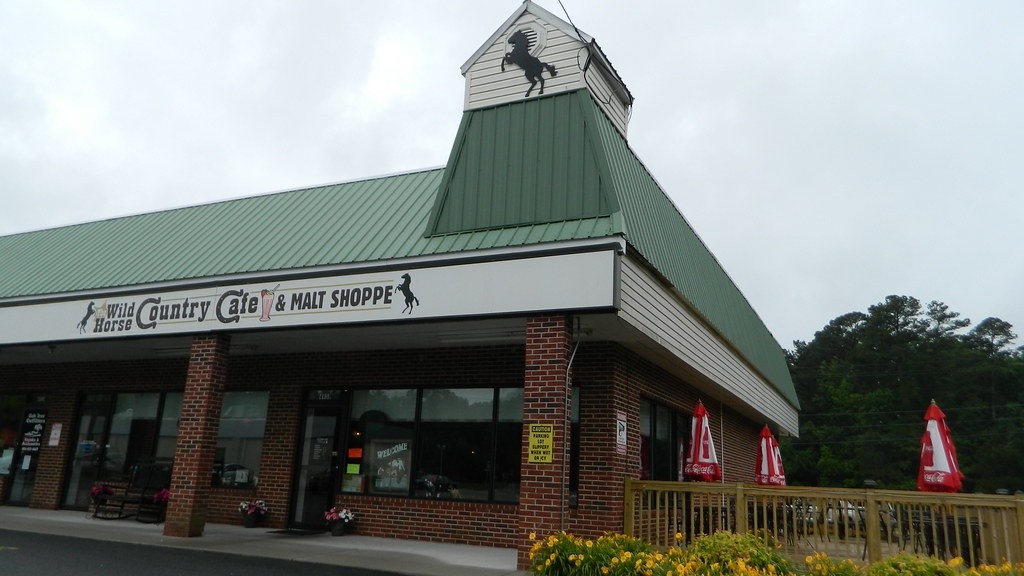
[447,482,465,499]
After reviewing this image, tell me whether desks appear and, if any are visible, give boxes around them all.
[911,515,987,568]
[830,506,857,540]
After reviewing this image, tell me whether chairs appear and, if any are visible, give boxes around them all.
[854,500,903,562]
[695,502,814,549]
[894,504,925,554]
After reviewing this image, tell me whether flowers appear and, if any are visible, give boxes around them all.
[92,482,113,495]
[238,492,269,516]
[154,488,169,501]
[323,507,356,522]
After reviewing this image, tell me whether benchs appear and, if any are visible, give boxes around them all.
[93,458,175,523]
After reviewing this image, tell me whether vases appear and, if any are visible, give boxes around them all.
[91,492,108,505]
[329,520,346,536]
[243,512,258,527]
[160,501,167,513]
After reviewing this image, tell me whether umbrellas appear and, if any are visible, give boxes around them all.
[916,398,966,493]
[682,399,721,482]
[753,423,786,486]
